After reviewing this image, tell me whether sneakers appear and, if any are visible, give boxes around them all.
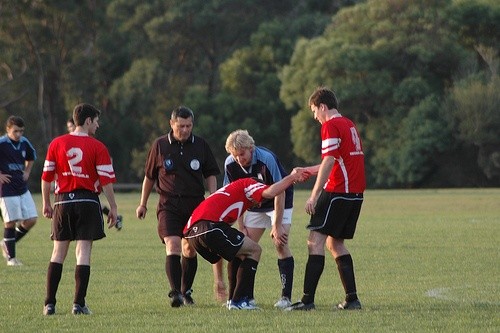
[43,304,55,315]
[6,258,24,266]
[283,301,316,312]
[72,304,93,316]
[168,288,183,307]
[226,297,258,310]
[274,297,293,308]
[181,289,197,307]
[331,298,361,310]
[0,239,12,260]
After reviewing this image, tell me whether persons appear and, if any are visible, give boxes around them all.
[223,129,295,309]
[136,105,222,308]
[41,103,118,315]
[282,88,366,312]
[183,167,311,311]
[66,118,123,231]
[0,115,37,267]
